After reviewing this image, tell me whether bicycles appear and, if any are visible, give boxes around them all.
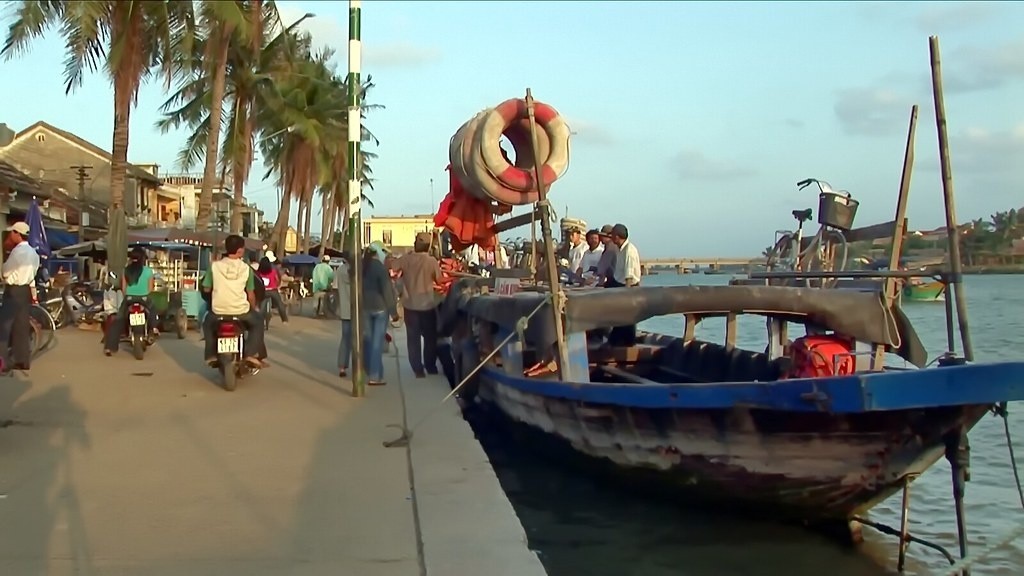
[762,177,860,291]
[261,272,283,331]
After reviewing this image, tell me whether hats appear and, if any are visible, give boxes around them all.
[321,255,331,260]
[564,225,581,234]
[606,224,628,239]
[368,240,392,253]
[5,221,31,235]
[415,232,431,244]
[598,225,613,238]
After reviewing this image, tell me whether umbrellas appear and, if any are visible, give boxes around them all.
[60,240,106,259]
[24,196,52,286]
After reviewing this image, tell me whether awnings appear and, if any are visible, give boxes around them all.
[41,227,80,250]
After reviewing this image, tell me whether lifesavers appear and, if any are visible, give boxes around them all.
[448,98,572,208]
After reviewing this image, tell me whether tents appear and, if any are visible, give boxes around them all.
[103,227,266,289]
[281,254,347,265]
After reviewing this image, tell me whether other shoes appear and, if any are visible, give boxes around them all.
[205,356,217,367]
[104,348,113,357]
[339,367,346,378]
[1,368,10,376]
[416,364,438,378]
[152,327,161,338]
[10,363,30,370]
[259,358,270,368]
[368,380,387,386]
[245,356,262,369]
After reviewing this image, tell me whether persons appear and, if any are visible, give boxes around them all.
[393,232,463,378]
[0,222,40,375]
[103,248,161,357]
[200,235,270,368]
[524,224,641,377]
[312,231,400,387]
[250,251,312,319]
[35,258,110,292]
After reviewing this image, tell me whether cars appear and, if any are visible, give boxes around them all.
[326,257,349,275]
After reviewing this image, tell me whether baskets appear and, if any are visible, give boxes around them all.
[817,191,860,231]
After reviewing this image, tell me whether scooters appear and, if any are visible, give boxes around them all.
[0,260,106,355]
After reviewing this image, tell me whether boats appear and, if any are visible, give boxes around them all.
[434,264,1024,522]
[729,261,946,302]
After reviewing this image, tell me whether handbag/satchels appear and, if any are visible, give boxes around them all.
[103,285,124,316]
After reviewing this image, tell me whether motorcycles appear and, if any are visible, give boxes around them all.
[127,240,214,340]
[121,289,163,360]
[204,287,270,391]
